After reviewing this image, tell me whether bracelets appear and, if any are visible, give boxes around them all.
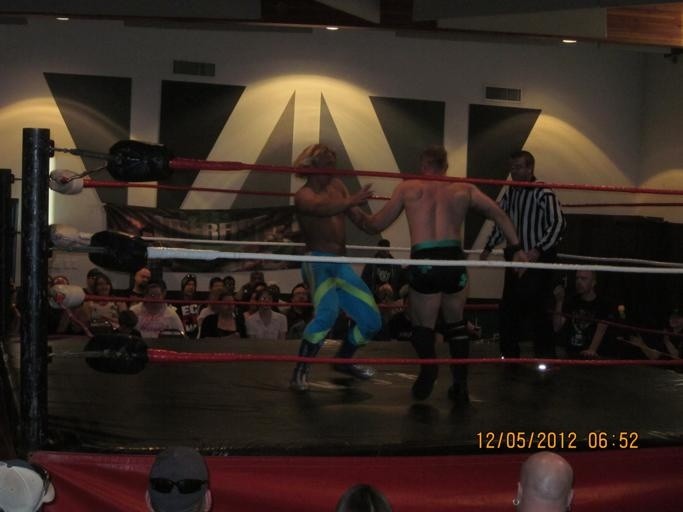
[510,242,522,254]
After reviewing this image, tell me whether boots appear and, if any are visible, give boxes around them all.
[410,326,438,400]
[332,339,373,380]
[447,338,470,407]
[290,339,321,394]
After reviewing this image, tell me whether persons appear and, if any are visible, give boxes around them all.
[335,483,393,512]
[289,142,382,393]
[512,450,575,512]
[479,150,566,379]
[360,144,530,402]
[144,445,212,512]
[9,268,149,375]
[543,269,683,360]
[129,271,314,338]
[360,239,416,339]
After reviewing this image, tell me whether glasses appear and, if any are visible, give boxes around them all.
[150,478,206,493]
